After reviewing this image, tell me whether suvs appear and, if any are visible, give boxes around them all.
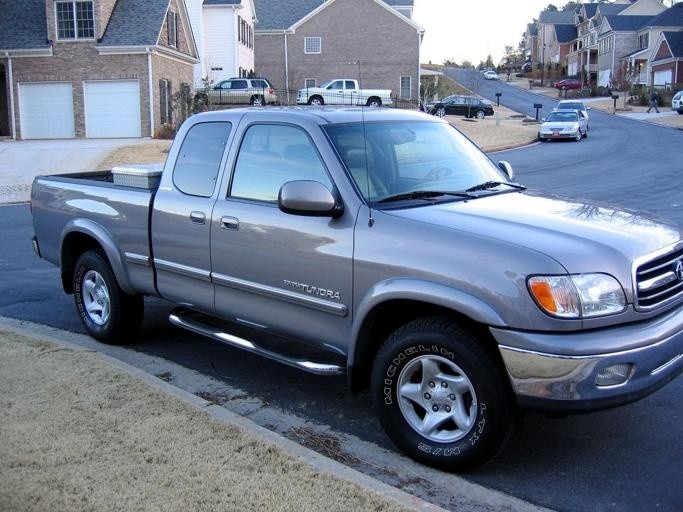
[193,76,280,107]
[552,99,592,128]
[521,62,532,72]
[671,90,682,114]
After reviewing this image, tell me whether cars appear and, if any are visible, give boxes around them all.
[427,95,494,118]
[536,109,588,141]
[483,71,499,81]
[553,79,590,90]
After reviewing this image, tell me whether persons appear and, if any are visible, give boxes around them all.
[645,89,659,113]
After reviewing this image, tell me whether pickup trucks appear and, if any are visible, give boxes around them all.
[295,78,395,108]
[28,105,683,472]
